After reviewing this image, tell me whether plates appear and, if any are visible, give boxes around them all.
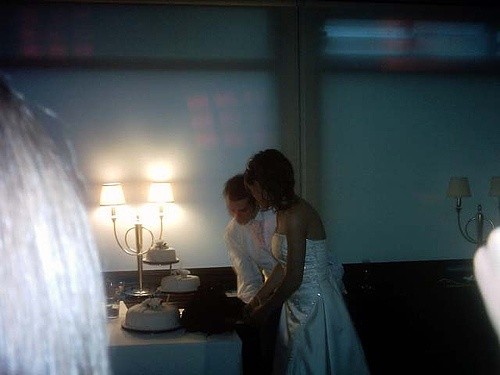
[156,286,198,296]
[120,322,181,332]
[143,259,180,265]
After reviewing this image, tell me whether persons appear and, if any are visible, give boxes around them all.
[224,174,291,375]
[473,228,500,339]
[0,77,111,375]
[243,149,370,375]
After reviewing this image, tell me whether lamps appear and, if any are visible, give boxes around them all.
[448,176,500,280]
[100,183,174,297]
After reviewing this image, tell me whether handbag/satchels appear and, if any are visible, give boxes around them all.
[178,283,245,337]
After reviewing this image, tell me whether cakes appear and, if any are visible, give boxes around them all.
[145,241,176,262]
[161,269,200,292]
[125,297,180,330]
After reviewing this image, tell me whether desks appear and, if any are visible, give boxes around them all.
[108,309,242,375]
